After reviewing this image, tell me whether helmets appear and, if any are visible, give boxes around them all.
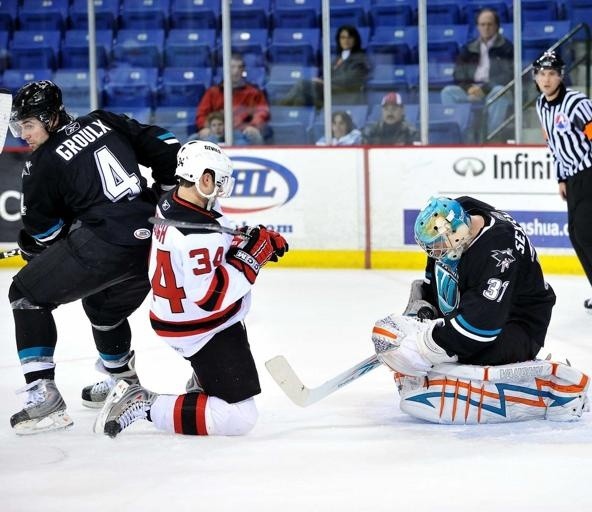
[414,197,471,259]
[10,80,73,141]
[175,139,235,208]
[533,52,565,77]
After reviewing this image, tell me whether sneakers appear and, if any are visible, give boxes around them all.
[187,369,203,393]
[10,353,156,438]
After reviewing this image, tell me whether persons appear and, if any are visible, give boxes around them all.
[92,140,288,440]
[9,79,182,434]
[198,112,249,146]
[533,51,592,308]
[438,6,516,145]
[197,52,270,132]
[357,93,420,146]
[372,196,592,423]
[278,25,371,105]
[314,111,362,145]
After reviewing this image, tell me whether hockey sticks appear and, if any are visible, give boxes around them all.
[263,353,380,408]
[149,216,241,236]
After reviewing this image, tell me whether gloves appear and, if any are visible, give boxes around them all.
[225,223,288,284]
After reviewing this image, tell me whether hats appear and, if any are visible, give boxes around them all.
[381,93,404,106]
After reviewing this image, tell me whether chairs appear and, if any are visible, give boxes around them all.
[0,1,592,148]
